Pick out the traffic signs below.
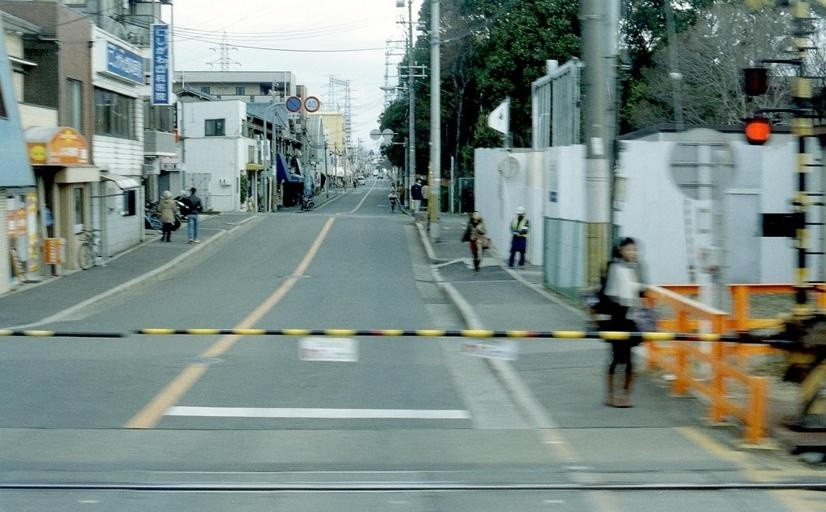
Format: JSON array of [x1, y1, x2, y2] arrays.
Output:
[[304, 96, 319, 112]]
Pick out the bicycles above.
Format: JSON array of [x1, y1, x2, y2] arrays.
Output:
[[76, 229, 104, 270]]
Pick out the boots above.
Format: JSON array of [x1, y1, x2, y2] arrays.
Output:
[[473, 259, 481, 272], [160, 227, 172, 242], [606, 373, 634, 408]]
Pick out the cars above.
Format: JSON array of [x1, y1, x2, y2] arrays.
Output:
[[373, 169, 383, 179]]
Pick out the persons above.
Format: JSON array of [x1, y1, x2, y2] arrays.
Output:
[[463, 211, 490, 271], [184, 188, 204, 243], [389, 191, 397, 213], [399, 183, 404, 205], [508, 206, 530, 267], [158, 191, 178, 242], [589, 236, 650, 409], [411, 178, 424, 211], [353, 181, 356, 189]]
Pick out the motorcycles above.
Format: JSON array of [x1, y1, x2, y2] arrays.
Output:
[[300, 195, 315, 210]]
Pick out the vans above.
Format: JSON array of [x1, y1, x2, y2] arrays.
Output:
[[357, 176, 365, 185]]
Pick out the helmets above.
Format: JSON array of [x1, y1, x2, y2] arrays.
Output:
[[515, 206, 526, 214]]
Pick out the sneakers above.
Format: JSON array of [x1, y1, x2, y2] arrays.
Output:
[[187, 238, 200, 244]]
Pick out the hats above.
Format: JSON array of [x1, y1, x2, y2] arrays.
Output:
[[162, 190, 171, 199]]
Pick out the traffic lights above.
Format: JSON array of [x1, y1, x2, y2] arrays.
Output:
[[745, 118, 770, 145], [744, 68, 768, 97]]
